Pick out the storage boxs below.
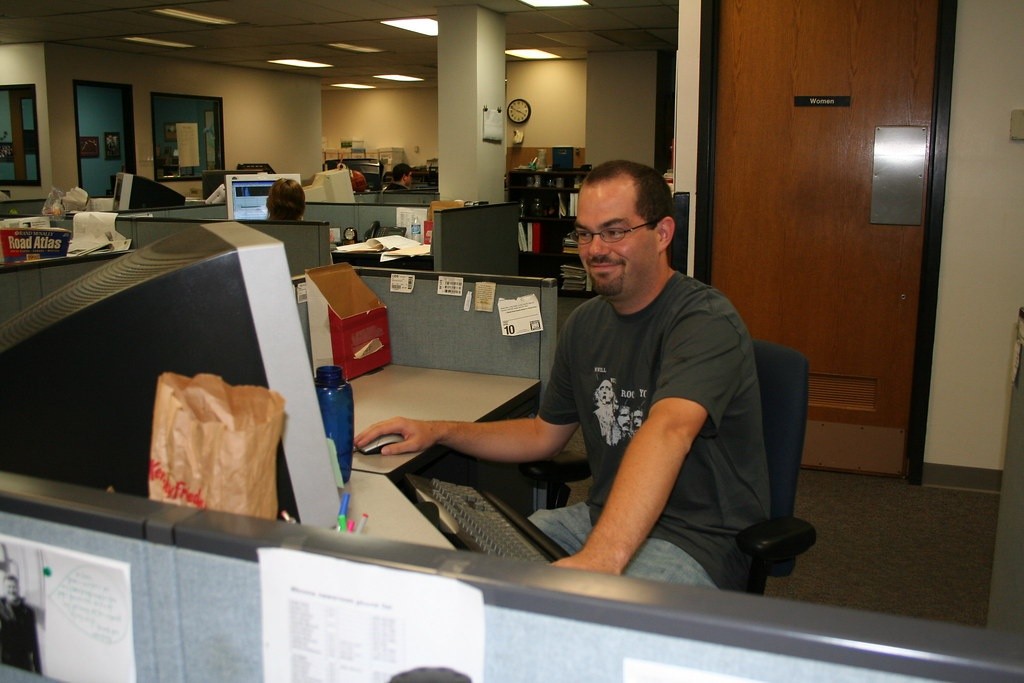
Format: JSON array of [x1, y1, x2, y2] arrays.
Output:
[[322, 137, 404, 172], [304, 263, 391, 381], [424, 200, 463, 244]]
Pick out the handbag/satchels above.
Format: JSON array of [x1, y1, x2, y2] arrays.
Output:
[[149, 371, 285, 520]]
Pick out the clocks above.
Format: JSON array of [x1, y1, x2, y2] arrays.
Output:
[[507, 99, 531, 124]]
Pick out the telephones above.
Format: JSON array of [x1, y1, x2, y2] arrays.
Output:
[[365, 221, 406, 240]]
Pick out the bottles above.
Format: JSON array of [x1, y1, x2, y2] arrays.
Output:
[[90, 200, 95, 211], [411, 216, 421, 243], [527, 177, 534, 186], [556, 178, 563, 187], [536, 149, 547, 169]]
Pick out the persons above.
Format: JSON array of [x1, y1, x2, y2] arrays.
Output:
[[0, 576, 43, 676], [266, 178, 306, 221], [107, 136, 117, 154], [387, 163, 413, 190], [353, 160, 771, 593]]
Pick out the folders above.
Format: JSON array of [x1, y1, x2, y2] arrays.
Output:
[[518, 222, 540, 252]]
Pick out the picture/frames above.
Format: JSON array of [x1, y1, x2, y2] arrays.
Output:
[[104, 132, 120, 159], [79, 137, 99, 157], [164, 122, 177, 141], [0, 142, 14, 161]]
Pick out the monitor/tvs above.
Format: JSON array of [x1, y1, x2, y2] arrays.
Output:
[[0, 168, 355, 532]]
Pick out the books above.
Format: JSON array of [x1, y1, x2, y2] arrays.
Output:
[[560, 264, 592, 291], [518, 222, 542, 253], [562, 238, 579, 254], [570, 193, 578, 216]]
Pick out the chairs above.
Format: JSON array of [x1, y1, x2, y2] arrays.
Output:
[[518, 339, 816, 596]]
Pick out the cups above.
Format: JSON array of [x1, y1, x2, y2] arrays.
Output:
[[313, 365, 354, 484], [518, 166, 529, 169]]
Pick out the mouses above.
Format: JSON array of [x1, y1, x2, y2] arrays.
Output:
[[357, 433, 404, 454]]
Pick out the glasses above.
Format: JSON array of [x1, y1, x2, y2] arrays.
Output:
[[567, 215, 667, 245]]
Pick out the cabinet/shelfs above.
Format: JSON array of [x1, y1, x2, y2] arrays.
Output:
[[508, 166, 591, 291]]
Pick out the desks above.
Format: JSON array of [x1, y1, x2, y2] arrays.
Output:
[[338, 364, 542, 550]]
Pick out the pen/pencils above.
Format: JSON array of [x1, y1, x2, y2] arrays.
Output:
[[531, 155, 537, 165], [335, 491, 371, 533], [280, 510, 292, 520]]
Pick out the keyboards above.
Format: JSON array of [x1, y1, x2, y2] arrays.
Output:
[[403, 473, 551, 565]]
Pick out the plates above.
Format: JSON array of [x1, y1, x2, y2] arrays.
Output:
[[514, 168, 532, 171]]
